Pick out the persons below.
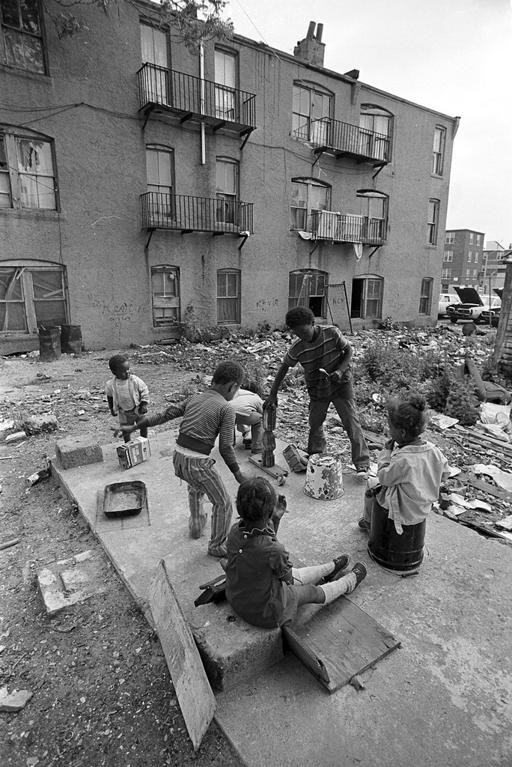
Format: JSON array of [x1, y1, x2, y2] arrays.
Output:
[[104, 354, 151, 443], [228, 378, 266, 453], [109, 360, 248, 559], [358, 392, 452, 536], [262, 304, 372, 476], [224, 474, 368, 629]]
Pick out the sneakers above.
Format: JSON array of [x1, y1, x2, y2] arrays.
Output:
[[358, 519, 372, 535], [325, 552, 353, 584], [356, 464, 371, 478], [343, 560, 368, 594]]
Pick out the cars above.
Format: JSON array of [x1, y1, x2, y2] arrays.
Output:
[[438, 286, 501, 324]]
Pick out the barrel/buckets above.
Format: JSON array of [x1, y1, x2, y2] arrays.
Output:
[[304, 452, 345, 501], [61, 323, 83, 355], [39, 326, 61, 360], [367, 503, 430, 577]]
